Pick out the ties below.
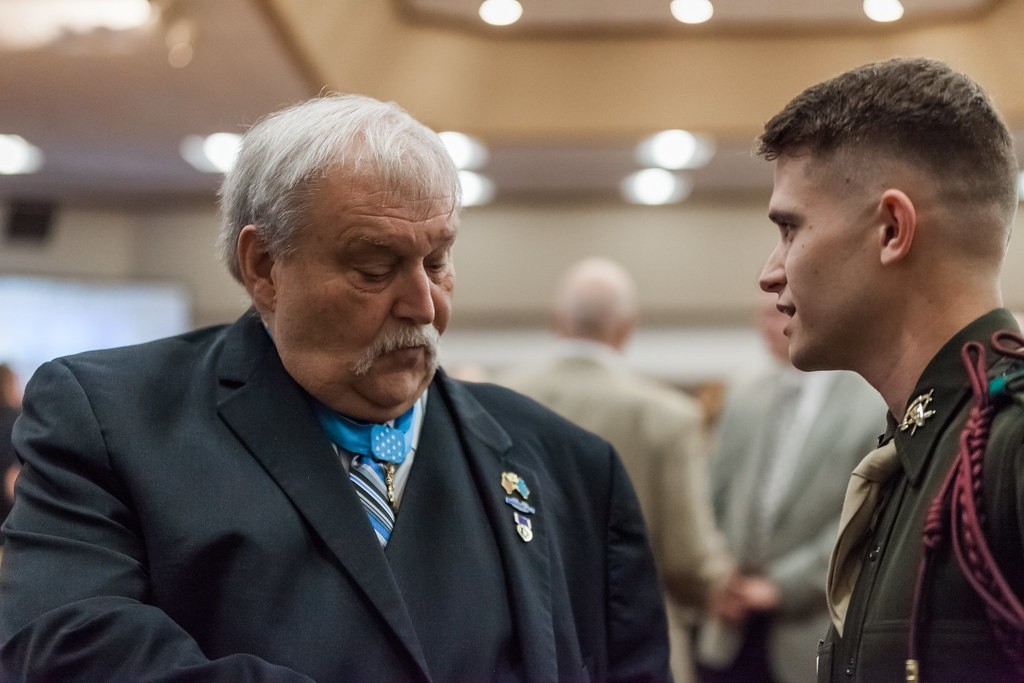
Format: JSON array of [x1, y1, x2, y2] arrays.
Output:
[[349, 452, 398, 551], [824, 436, 899, 636]]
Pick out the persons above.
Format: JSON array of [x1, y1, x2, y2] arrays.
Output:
[[521, 258, 888, 683], [749, 56, 1024, 683], [0, 93, 675, 683], [0, 363, 29, 533]]
